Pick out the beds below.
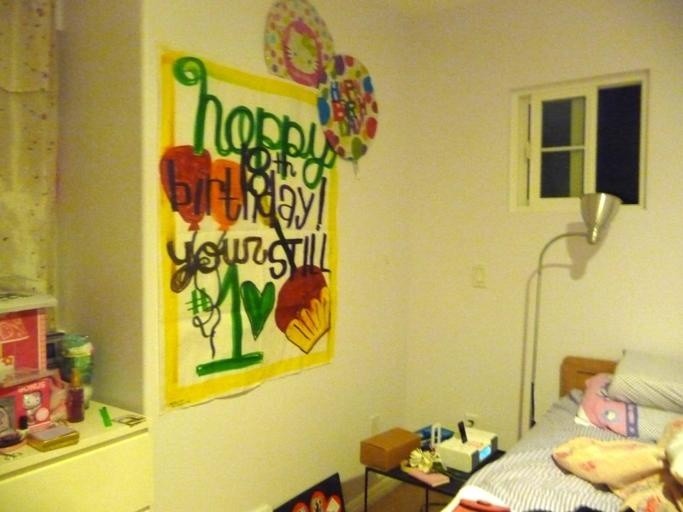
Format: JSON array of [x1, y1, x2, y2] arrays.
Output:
[[441, 346, 683, 512]]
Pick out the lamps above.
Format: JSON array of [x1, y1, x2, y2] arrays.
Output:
[[530, 193, 622, 429]]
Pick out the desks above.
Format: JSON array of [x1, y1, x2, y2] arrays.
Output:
[[364, 423, 506, 512]]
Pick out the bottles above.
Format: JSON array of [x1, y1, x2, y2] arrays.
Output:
[[68, 367, 86, 422]]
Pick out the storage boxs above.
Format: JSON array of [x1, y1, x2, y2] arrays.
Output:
[[360, 427, 421, 471]]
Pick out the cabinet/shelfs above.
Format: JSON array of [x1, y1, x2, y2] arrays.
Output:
[[0, 398, 156, 512]]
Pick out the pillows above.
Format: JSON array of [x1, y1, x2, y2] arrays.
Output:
[[573, 372, 682, 440], [605, 349, 682, 414]]
